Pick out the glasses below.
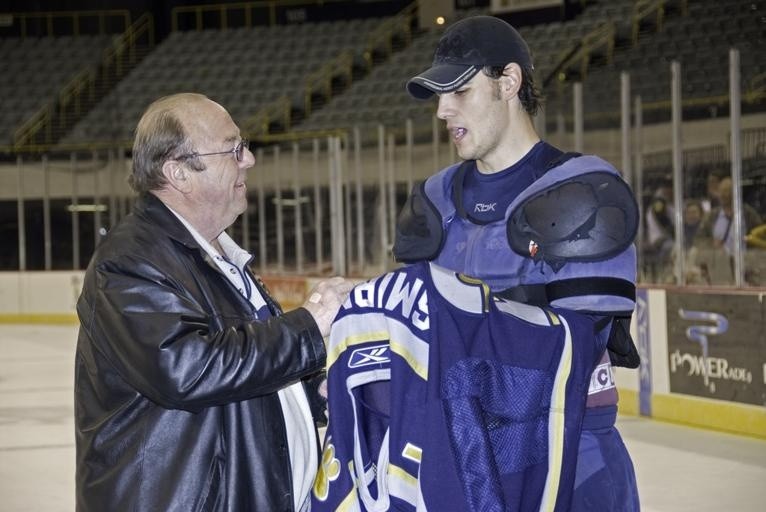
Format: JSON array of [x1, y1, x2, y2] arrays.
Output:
[[175, 137, 249, 162]]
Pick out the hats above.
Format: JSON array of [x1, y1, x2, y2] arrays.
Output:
[[408, 14, 533, 99]]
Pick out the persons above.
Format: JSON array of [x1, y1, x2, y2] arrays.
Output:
[[306, 16, 640, 512], [642, 162, 766, 287], [71, 90, 356, 511]]
